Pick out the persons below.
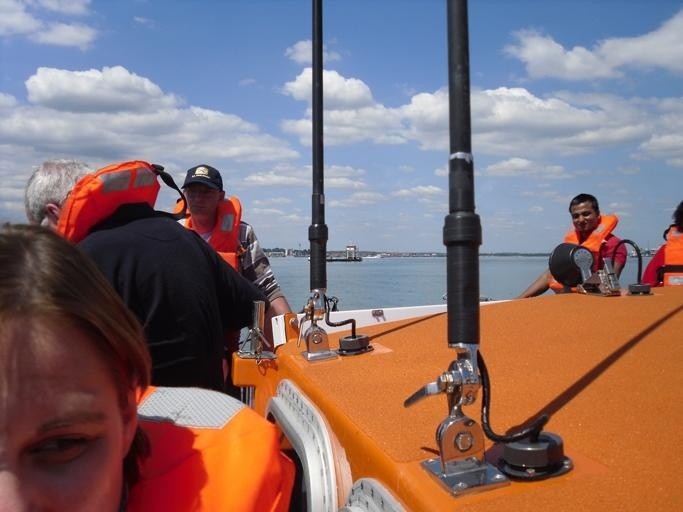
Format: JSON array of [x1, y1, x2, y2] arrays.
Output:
[[641, 200, 683, 287], [172, 164, 293, 320], [26, 157, 271, 392], [0, 222, 299, 511], [517, 192, 628, 299]]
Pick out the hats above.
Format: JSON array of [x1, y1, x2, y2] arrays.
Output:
[[181, 164, 223, 190]]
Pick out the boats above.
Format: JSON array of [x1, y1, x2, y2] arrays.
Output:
[[228, 284, 681, 512]]
